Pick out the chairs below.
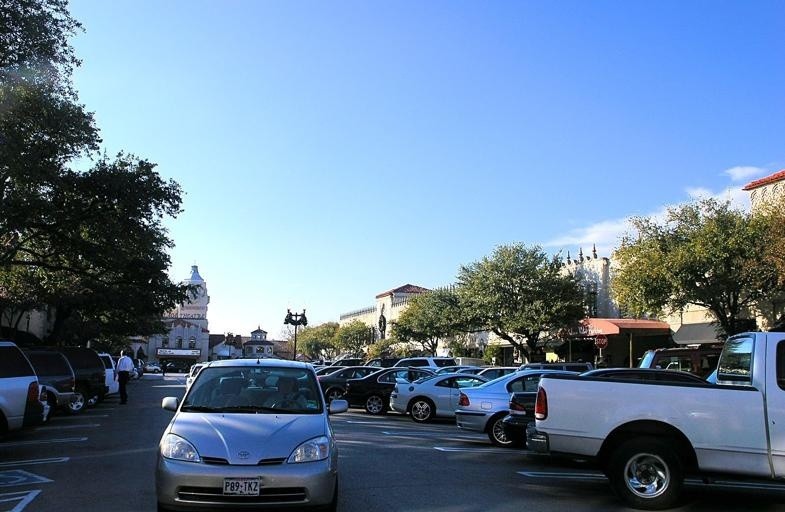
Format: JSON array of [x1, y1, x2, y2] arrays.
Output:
[[205, 376, 312, 407]]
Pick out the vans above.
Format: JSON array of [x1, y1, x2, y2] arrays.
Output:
[[636, 343, 726, 380]]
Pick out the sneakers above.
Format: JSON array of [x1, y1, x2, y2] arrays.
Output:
[[119, 400, 128, 405]]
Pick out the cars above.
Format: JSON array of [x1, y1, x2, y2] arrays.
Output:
[[0, 338, 145, 441], [501, 365, 715, 473], [144, 362, 161, 374]]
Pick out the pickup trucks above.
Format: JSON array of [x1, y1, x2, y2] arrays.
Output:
[[522, 330, 785, 511]]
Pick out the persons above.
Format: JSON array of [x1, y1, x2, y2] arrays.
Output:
[[160, 361, 167, 377], [113, 348, 135, 406]]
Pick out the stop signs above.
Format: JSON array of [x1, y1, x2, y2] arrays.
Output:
[[593, 333, 608, 349]]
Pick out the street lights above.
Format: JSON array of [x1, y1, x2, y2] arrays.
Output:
[[283, 308, 307, 359]]
